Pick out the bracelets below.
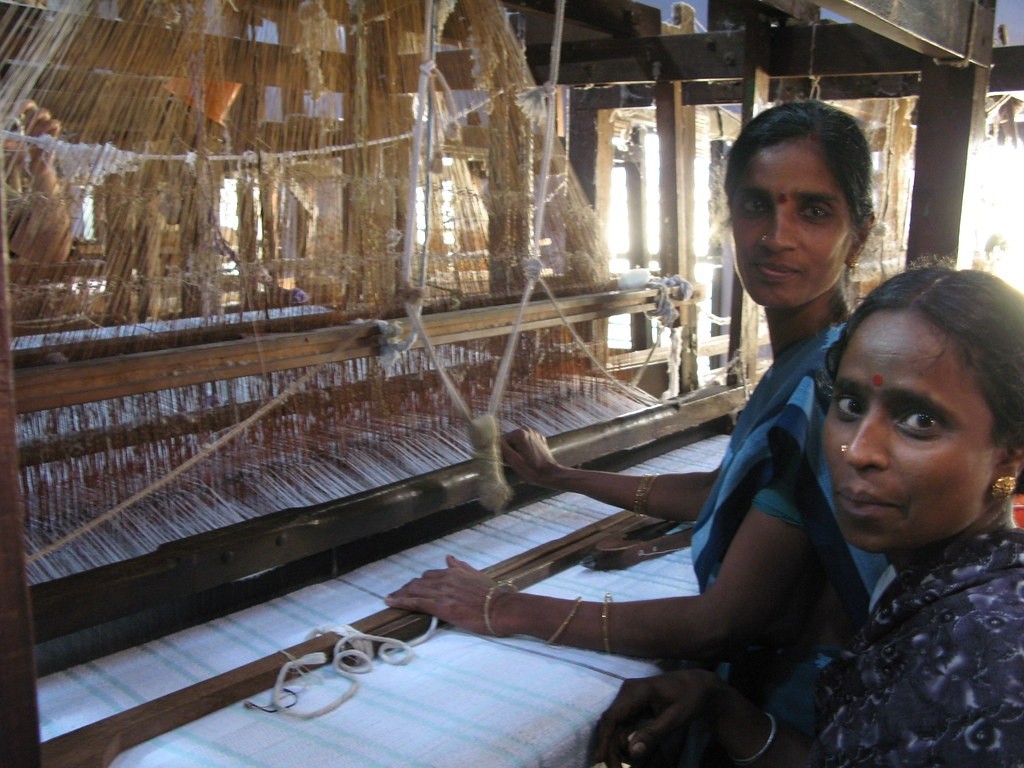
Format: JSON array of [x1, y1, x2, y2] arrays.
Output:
[[601, 594, 613, 655], [634, 473, 660, 517], [483, 580, 517, 638], [544, 596, 582, 645], [730, 711, 777, 766]]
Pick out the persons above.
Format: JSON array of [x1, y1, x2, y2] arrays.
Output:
[[383, 103, 894, 666], [0, 100, 72, 265], [584, 266, 1024, 768]]
